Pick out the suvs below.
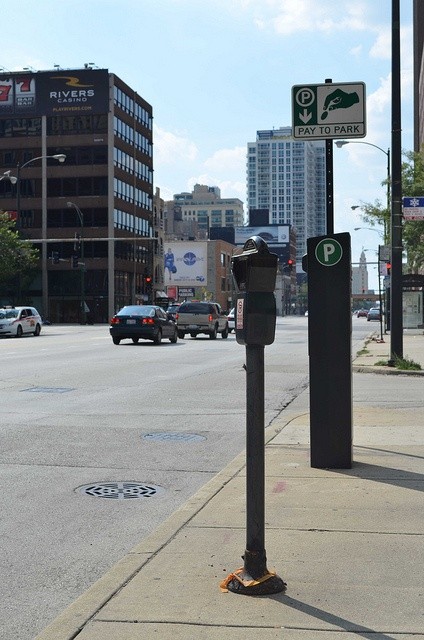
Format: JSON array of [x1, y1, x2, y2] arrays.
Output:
[[175, 300, 228, 339]]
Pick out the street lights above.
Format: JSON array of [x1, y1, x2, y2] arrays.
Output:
[[351, 205, 386, 331], [334, 141, 390, 334], [66, 202, 85, 324], [16, 154, 66, 307]]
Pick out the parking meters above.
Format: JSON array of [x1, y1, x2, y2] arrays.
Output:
[[225, 235, 287, 595]]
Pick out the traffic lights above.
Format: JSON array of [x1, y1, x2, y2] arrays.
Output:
[[386, 263, 391, 274], [362, 287, 365, 293], [144, 267, 152, 282], [287, 260, 293, 271]]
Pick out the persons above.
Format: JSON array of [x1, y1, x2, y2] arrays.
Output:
[[165, 248, 174, 281]]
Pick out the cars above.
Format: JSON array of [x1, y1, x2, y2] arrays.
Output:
[[228, 307, 236, 332], [109, 305, 178, 345], [367, 310, 381, 321], [357, 310, 367, 318], [0, 305, 42, 337]]
